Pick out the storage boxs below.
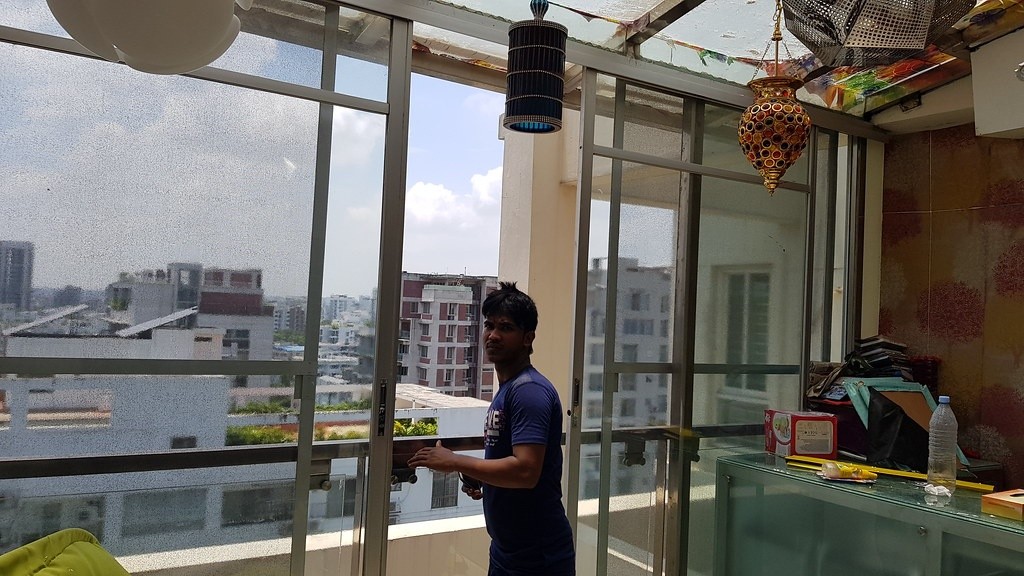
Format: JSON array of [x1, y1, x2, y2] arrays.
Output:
[[764, 410, 838, 459]]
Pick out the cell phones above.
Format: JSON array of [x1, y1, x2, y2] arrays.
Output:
[[462, 473, 481, 494]]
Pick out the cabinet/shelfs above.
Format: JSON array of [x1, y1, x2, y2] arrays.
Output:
[[714, 453, 1024, 576]]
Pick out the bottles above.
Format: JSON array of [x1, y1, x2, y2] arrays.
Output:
[[927, 396, 958, 495]]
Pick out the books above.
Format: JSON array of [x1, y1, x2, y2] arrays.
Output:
[[858, 334, 913, 376]]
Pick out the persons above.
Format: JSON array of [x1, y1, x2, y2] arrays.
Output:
[[407, 283, 577, 576]]
